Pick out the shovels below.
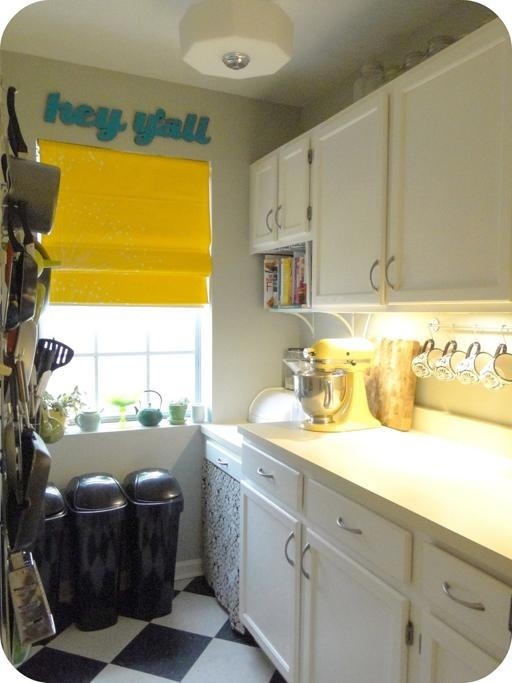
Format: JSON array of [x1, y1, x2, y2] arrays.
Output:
[[32, 338, 74, 417]]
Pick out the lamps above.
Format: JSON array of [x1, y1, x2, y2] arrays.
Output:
[[176, 0, 295, 81]]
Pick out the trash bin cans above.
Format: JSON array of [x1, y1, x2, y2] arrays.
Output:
[[30, 468, 185, 632]]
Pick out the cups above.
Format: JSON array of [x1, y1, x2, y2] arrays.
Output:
[[168, 420, 187, 425], [169, 405, 188, 419], [411, 339, 512, 391], [192, 406, 205, 424]]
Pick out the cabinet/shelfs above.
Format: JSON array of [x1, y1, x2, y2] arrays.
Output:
[[414, 510, 512, 683], [247, 133, 314, 254], [238, 424, 413, 682], [311, 15, 512, 307], [200, 425, 242, 633]]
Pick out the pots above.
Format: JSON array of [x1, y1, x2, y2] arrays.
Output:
[[1, 97, 61, 667]]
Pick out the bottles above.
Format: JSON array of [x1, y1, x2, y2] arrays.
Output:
[[284, 348, 304, 391]]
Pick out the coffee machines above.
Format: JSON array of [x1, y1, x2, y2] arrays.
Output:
[[296, 338, 386, 433]]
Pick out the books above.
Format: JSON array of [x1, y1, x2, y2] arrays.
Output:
[[262, 244, 306, 308]]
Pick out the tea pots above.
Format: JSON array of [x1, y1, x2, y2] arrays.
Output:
[[133, 389, 163, 426], [75, 408, 105, 433]]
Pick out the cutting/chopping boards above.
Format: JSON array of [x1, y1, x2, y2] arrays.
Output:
[[373, 337, 420, 433]]
